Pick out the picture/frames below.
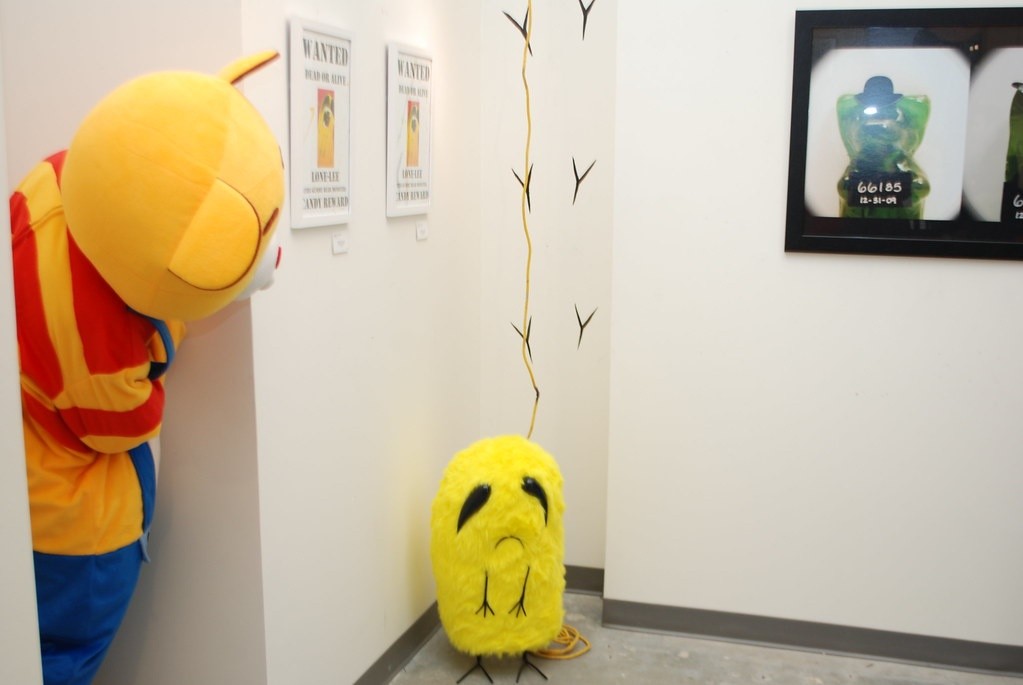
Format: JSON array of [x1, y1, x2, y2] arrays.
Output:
[[386, 46, 435, 218], [286, 19, 354, 231], [785, 7, 1023, 260]]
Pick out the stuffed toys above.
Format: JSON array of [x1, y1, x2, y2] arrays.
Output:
[[432, 433, 566, 654], [4, 49, 289, 685]]
